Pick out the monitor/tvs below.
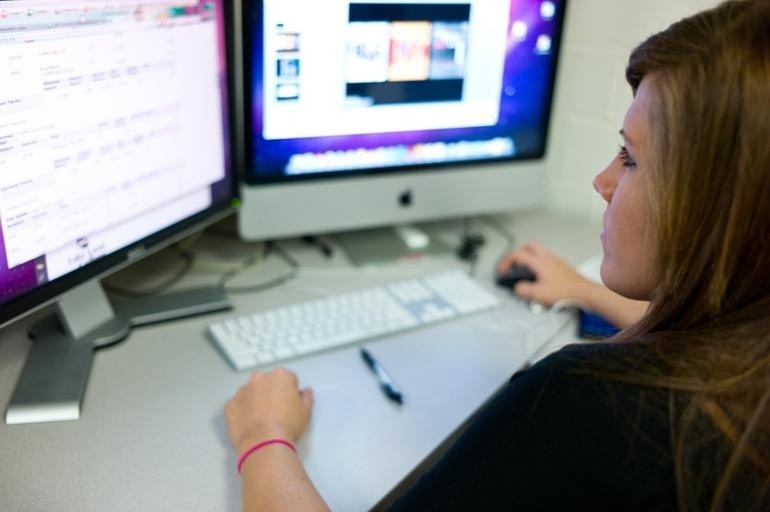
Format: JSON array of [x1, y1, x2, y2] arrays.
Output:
[[0, 1, 238, 423], [238, 1, 571, 267]]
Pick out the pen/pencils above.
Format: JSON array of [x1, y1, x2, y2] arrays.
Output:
[[360, 347, 402, 404]]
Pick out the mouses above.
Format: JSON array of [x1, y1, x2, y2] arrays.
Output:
[[495, 265, 536, 289]]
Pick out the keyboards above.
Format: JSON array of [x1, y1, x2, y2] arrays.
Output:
[[206, 270, 502, 373]]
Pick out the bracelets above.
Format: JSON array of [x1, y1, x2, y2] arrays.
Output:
[[238, 437, 296, 473]]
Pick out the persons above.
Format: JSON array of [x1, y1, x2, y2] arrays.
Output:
[[220, 0, 770, 512]]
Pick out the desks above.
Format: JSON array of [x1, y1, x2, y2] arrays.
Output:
[[1, 203, 603, 512]]
